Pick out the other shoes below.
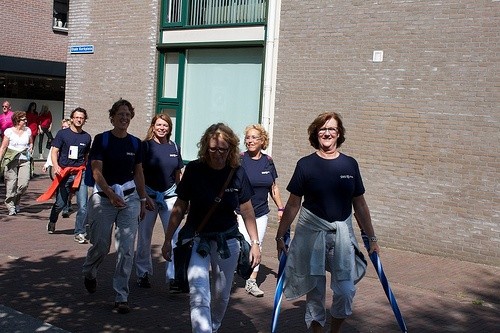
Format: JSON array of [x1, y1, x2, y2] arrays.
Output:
[[46, 222, 55, 234], [68, 205, 73, 212], [83, 276, 97, 294], [245, 279, 264, 297], [115, 301, 130, 314], [137, 272, 150, 284], [62, 210, 69, 217]]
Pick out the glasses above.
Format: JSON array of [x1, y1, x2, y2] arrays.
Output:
[[1, 105, 9, 108], [115, 110, 133, 118], [206, 146, 231, 154], [18, 117, 28, 122], [316, 126, 338, 135]]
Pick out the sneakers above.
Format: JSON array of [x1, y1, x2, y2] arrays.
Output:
[[74, 234, 87, 244], [15, 205, 21, 213], [169, 281, 182, 294], [8, 208, 17, 216]]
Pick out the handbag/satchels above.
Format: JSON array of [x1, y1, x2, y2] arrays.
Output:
[[173, 240, 194, 294], [1, 148, 23, 171]]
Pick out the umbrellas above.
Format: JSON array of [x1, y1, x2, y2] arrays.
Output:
[[271, 228, 290, 333], [353, 212, 408, 333]]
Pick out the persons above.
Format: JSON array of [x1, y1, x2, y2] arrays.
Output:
[[25, 101, 53, 159], [83, 100, 146, 311], [233, 124, 284, 296], [0, 100, 14, 138], [42, 118, 73, 218], [36, 108, 92, 243], [0, 111, 33, 215], [162, 123, 261, 333], [136, 113, 183, 292], [276, 112, 379, 333]]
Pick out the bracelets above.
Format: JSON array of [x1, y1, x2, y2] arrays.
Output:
[[251, 240, 260, 245], [278, 209, 284, 211], [370, 237, 377, 241], [140, 198, 146, 201], [276, 237, 284, 241]]
